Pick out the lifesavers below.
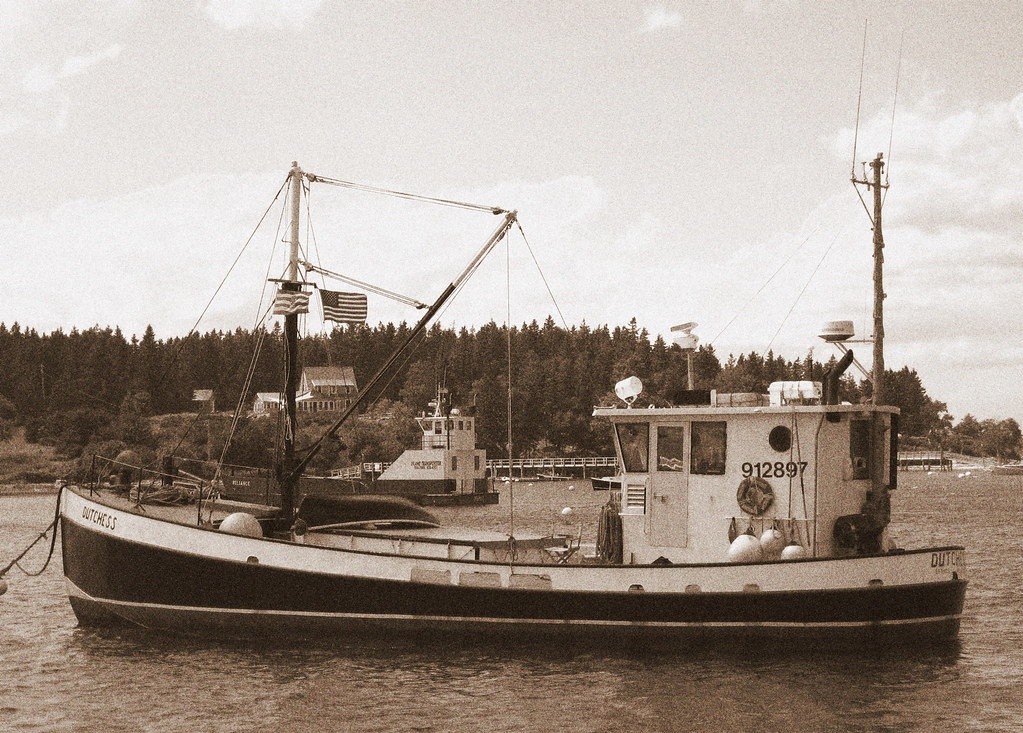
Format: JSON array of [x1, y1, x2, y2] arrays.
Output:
[[736, 476, 775, 517]]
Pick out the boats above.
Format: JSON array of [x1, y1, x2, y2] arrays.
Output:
[[57, 161, 970, 655], [590, 476, 621, 491], [370, 368, 501, 510]]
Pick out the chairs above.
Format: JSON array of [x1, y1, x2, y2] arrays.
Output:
[[543, 526, 583, 567]]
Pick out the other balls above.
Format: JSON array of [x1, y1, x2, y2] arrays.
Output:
[[219, 513, 263, 537]]
